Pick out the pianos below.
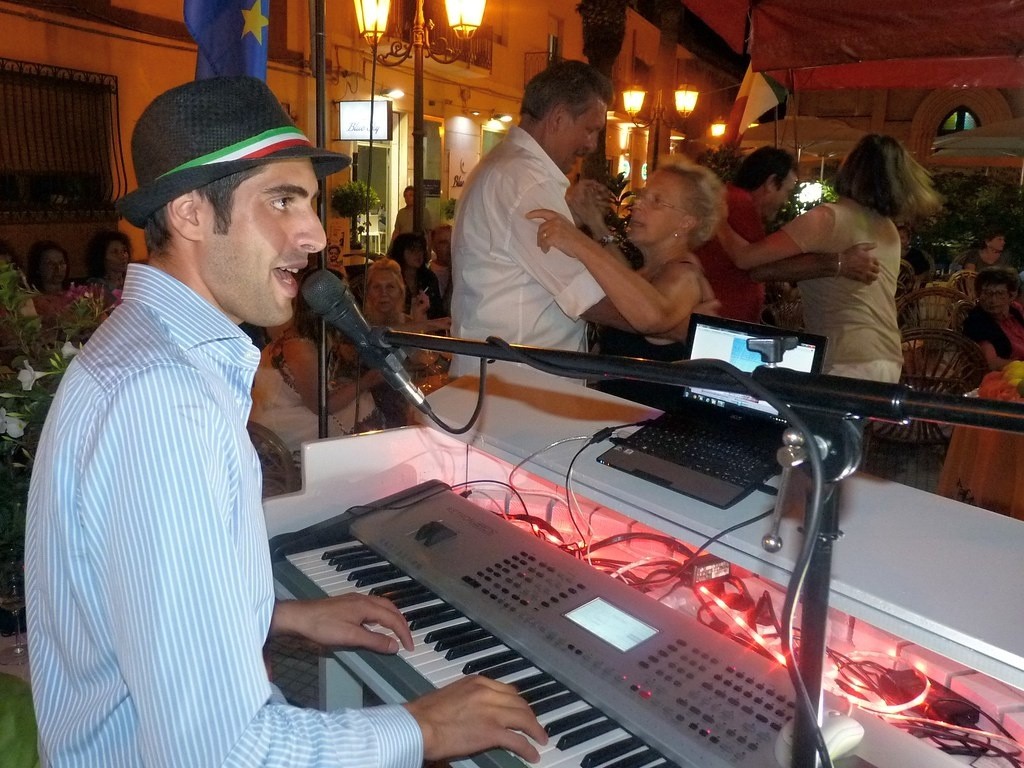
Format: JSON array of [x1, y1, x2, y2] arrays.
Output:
[[267, 476, 868, 767]]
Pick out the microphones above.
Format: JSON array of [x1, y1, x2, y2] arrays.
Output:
[[299, 270, 433, 414]]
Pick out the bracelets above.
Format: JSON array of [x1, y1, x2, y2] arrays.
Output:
[[835, 253, 841, 278]]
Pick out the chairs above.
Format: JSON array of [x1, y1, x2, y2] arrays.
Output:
[[244, 257, 1024, 500]]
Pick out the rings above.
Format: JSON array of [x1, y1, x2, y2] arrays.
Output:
[[544, 232, 547, 238]]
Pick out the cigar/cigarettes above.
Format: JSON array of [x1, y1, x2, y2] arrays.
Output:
[[423, 287, 428, 293]]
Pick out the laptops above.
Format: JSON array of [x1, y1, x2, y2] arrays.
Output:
[[598, 311, 828, 510]]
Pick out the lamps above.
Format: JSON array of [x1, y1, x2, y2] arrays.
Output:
[[381, 89, 403, 101], [489, 112, 512, 121]]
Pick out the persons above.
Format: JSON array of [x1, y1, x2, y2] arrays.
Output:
[[250, 187, 452, 466], [695, 146, 879, 329], [526, 154, 730, 361], [24, 78, 550, 768], [962, 264, 1024, 372], [897, 224, 930, 276], [27, 240, 70, 291], [84, 231, 130, 288], [963, 228, 1008, 292], [715, 133, 946, 384], [450, 58, 721, 388]]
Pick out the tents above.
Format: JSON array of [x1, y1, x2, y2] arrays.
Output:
[[680, 0, 1024, 165]]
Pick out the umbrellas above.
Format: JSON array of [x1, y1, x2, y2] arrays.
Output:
[[928, 116, 1024, 188], [740, 117, 870, 182]]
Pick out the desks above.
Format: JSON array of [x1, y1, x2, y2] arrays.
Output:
[[938, 360, 1024, 522]]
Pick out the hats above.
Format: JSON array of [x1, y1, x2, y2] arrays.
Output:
[[114, 75, 352, 228]]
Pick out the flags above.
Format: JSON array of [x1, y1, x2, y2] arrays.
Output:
[[723, 60, 787, 144], [183, 0, 269, 83]]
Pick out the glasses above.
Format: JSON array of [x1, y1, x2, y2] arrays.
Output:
[[631, 185, 691, 218], [982, 288, 1009, 298]]
